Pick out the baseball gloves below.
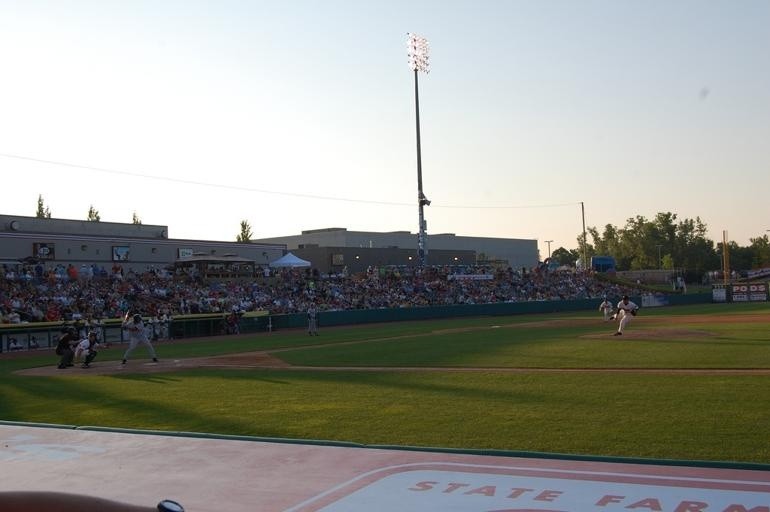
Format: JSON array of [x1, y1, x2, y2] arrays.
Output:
[[631, 309, 637, 316], [100, 343, 112, 349]]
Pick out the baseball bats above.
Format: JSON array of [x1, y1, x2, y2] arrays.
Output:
[[122, 310, 130, 327]]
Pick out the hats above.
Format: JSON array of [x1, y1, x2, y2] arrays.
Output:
[[134, 313, 142, 323], [622, 295, 630, 299], [68, 327, 76, 333]]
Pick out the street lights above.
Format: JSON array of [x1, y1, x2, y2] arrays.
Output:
[[406, 31, 433, 267]]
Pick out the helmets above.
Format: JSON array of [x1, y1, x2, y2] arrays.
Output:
[[88, 331, 98, 337]]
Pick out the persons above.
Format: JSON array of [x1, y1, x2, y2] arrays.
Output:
[[118, 307, 160, 365], [55, 327, 77, 369], [75, 331, 109, 369]]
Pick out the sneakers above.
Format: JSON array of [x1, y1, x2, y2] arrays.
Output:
[[57, 362, 90, 369], [123, 359, 126, 363], [615, 331, 622, 336], [153, 357, 159, 362]]
